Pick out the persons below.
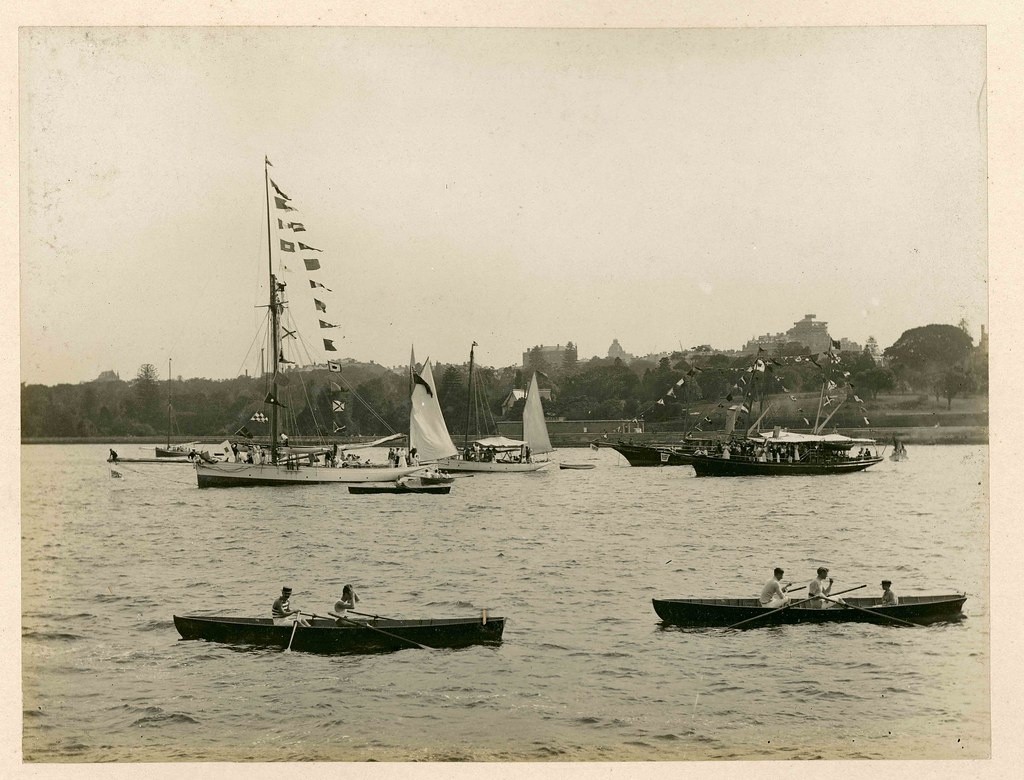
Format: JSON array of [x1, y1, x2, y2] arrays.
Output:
[[231, 442, 266, 465], [809, 567, 851, 608], [472, 443, 497, 462], [272, 587, 311, 627], [628, 437, 633, 445], [279, 433, 289, 446], [617, 437, 624, 445], [526, 446, 530, 463], [716, 439, 723, 454], [729, 437, 742, 452], [389, 446, 420, 467], [747, 440, 808, 462], [334, 584, 372, 627], [325, 450, 361, 469], [759, 568, 788, 608], [110, 448, 118, 459], [188, 449, 198, 460], [858, 447, 871, 459], [872, 580, 898, 606]]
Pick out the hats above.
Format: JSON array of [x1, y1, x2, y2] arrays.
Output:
[[880, 581, 893, 585], [282, 587, 292, 594]]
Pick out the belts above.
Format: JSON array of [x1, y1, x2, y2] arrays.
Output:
[[762, 603, 766, 604]]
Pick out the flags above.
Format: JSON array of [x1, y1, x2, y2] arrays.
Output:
[[234, 159, 346, 438]]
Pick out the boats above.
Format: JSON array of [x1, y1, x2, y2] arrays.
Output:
[[891, 451, 908, 463], [347, 486, 450, 494], [171, 611, 505, 652], [560, 464, 595, 469], [666, 596, 968, 625]]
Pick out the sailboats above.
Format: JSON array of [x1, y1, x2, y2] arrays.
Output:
[[155, 356, 196, 457], [394, 341, 475, 487], [590, 363, 886, 478], [496, 370, 550, 463], [437, 341, 552, 472], [107, 153, 432, 487]]
